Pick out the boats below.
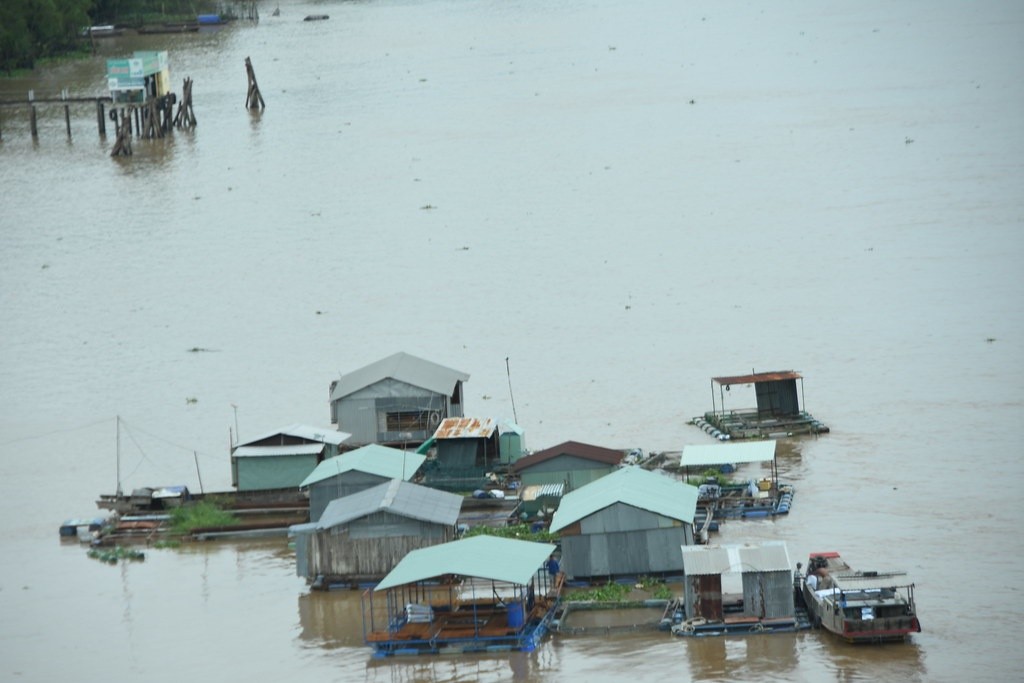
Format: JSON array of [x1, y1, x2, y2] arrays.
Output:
[[804, 551, 921, 642]]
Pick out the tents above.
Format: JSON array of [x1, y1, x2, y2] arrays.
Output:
[[711, 370, 805, 423], [680, 439, 777, 483], [374, 533, 557, 636]]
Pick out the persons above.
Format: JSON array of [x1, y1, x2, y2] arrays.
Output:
[[793, 562, 805, 600]]
